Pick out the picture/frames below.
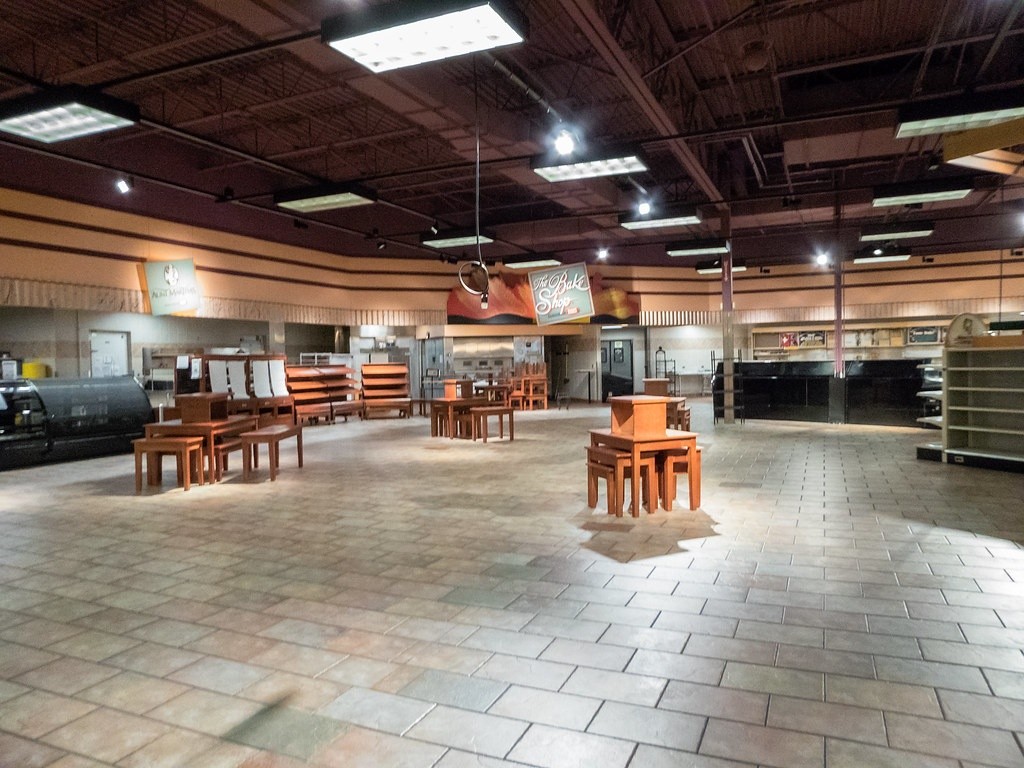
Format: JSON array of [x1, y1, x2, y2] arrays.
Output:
[[613, 348, 624, 363], [601, 348, 608, 363]]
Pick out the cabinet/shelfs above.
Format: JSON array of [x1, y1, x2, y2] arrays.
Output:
[[914, 347, 1024, 474], [711, 349, 745, 426]]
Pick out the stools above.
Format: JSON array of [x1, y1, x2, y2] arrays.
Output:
[[130, 391, 303, 492], [584, 378, 702, 519], [428, 363, 548, 443]]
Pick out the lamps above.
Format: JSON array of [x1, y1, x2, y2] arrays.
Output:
[[871, 173, 974, 209], [856, 220, 934, 242], [694, 258, 748, 276], [853, 247, 912, 264], [892, 91, 1024, 139], [420, 225, 495, 250], [0, 82, 142, 144], [532, 142, 654, 183], [665, 239, 731, 258], [274, 181, 381, 215], [618, 207, 704, 231], [501, 253, 563, 270], [320, 0, 531, 73]]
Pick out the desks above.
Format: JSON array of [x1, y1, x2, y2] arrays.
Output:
[[142, 414, 260, 487], [585, 428, 700, 518], [667, 396, 687, 430], [429, 396, 487, 439]]
